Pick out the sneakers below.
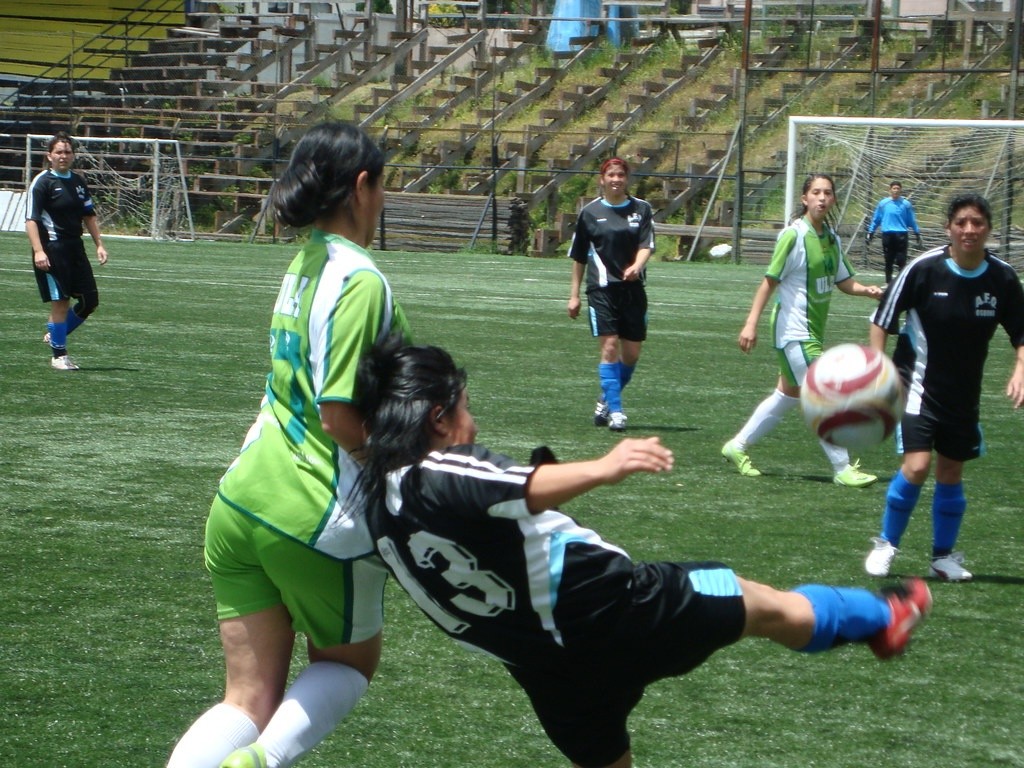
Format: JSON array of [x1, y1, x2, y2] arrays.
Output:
[[51, 354, 80, 371], [865, 535, 902, 577], [929, 551, 973, 581], [607, 410, 628, 430], [869, 576, 932, 661], [832, 458, 878, 489], [593, 400, 610, 424], [43, 333, 52, 344], [721, 440, 761, 477]]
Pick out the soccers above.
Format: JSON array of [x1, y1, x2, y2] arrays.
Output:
[[798, 343, 907, 450]]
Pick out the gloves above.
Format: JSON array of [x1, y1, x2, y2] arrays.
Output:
[[917, 233, 923, 245], [865, 232, 873, 246]]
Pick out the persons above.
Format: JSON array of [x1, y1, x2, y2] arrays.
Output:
[[337, 343, 931, 767], [165, 121, 416, 768], [866, 182, 923, 290], [720, 173, 884, 488], [24, 133, 108, 370], [567, 156, 655, 432], [865, 193, 1024, 579]]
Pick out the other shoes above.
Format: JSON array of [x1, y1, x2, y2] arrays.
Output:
[[880, 282, 892, 290]]
